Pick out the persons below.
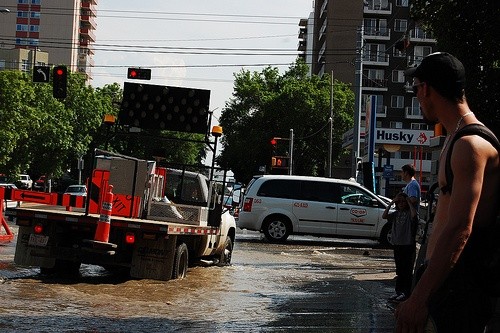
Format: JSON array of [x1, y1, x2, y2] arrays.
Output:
[[393, 52, 500, 333], [175, 182, 199, 200], [400, 164, 421, 220], [382, 192, 420, 302]]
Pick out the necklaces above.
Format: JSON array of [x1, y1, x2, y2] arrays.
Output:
[[439, 112, 474, 160]]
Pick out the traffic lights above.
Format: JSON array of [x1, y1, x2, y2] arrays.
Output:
[[53, 65, 67, 99], [127, 67, 152, 81], [276, 158, 289, 167], [271, 139, 278, 156]]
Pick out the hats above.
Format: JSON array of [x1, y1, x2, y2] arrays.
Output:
[[404, 52, 466, 89]]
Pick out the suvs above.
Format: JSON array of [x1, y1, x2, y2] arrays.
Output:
[[236, 174, 398, 249]]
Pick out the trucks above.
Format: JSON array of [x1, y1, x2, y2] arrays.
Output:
[[5, 115, 242, 282]]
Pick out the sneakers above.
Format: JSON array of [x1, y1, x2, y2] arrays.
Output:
[[388, 294, 401, 301], [394, 292, 411, 302]]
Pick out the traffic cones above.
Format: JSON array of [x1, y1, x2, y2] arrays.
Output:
[[83, 185, 117, 249]]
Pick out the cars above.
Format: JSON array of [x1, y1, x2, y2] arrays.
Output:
[[342, 193, 395, 209], [416, 194, 438, 244], [16, 174, 33, 190], [0, 183, 23, 212], [34, 176, 49, 189], [63, 184, 88, 196]]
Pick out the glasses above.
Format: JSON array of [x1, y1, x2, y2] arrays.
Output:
[[412, 82, 432, 95]]
